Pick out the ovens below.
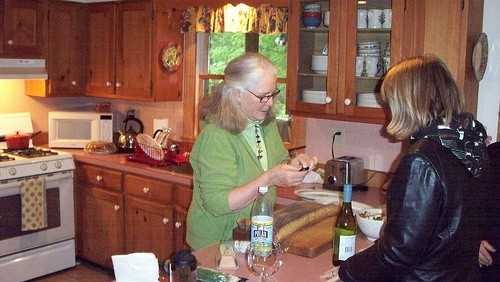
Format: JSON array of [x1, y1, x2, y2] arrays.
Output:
[[0, 169, 76, 282]]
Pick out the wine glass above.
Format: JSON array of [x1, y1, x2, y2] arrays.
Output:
[[247, 240, 283, 282]]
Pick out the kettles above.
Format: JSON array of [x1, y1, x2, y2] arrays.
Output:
[[117, 114, 144, 152]]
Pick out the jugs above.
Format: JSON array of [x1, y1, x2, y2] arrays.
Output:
[[276, 115, 292, 144], [164, 249, 201, 282], [153, 128, 176, 151], [357, 8, 392, 29]]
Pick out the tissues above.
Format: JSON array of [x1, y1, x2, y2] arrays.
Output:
[[110, 252, 170, 282]]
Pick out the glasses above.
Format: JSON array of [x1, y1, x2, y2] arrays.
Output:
[[245, 87, 280, 103]]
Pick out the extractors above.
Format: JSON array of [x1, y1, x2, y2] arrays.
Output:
[[0, 58, 48, 80]]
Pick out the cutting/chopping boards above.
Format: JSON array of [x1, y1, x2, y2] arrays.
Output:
[[272, 200, 342, 258]]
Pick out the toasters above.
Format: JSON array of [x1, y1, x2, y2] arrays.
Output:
[[324, 156, 364, 190]]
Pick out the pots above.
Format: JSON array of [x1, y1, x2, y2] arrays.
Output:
[[0, 132, 30, 150]]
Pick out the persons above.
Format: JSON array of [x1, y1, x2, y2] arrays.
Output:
[[477, 140, 500, 282], [185, 52, 318, 253], [320, 54, 489, 282]]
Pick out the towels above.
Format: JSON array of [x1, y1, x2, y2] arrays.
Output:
[[19, 176, 48, 231]]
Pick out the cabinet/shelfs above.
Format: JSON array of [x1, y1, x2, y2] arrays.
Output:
[[0, 0, 184, 102], [284, 0, 485, 126], [74, 160, 193, 274]]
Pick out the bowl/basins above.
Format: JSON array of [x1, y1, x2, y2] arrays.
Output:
[[355, 208, 386, 241], [356, 42, 390, 77], [302, 4, 322, 28]]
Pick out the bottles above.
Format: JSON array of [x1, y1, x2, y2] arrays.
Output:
[[332, 184, 357, 266], [250, 186, 274, 258], [233, 217, 251, 254]]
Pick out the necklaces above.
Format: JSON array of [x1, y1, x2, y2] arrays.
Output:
[[255, 125, 263, 160]]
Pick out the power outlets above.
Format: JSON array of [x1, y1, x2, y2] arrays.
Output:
[[328, 126, 345, 146]]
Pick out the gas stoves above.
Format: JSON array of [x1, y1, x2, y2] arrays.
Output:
[[0, 112, 76, 181]]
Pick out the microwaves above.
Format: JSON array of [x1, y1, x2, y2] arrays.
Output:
[[48, 109, 113, 148]]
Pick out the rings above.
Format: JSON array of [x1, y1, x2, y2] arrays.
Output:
[[332, 271, 334, 276]]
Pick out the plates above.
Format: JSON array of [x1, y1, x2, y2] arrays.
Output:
[[302, 90, 327, 104], [136, 134, 164, 161], [161, 41, 183, 72], [294, 189, 372, 212], [356, 92, 385, 107], [311, 44, 328, 74]]
